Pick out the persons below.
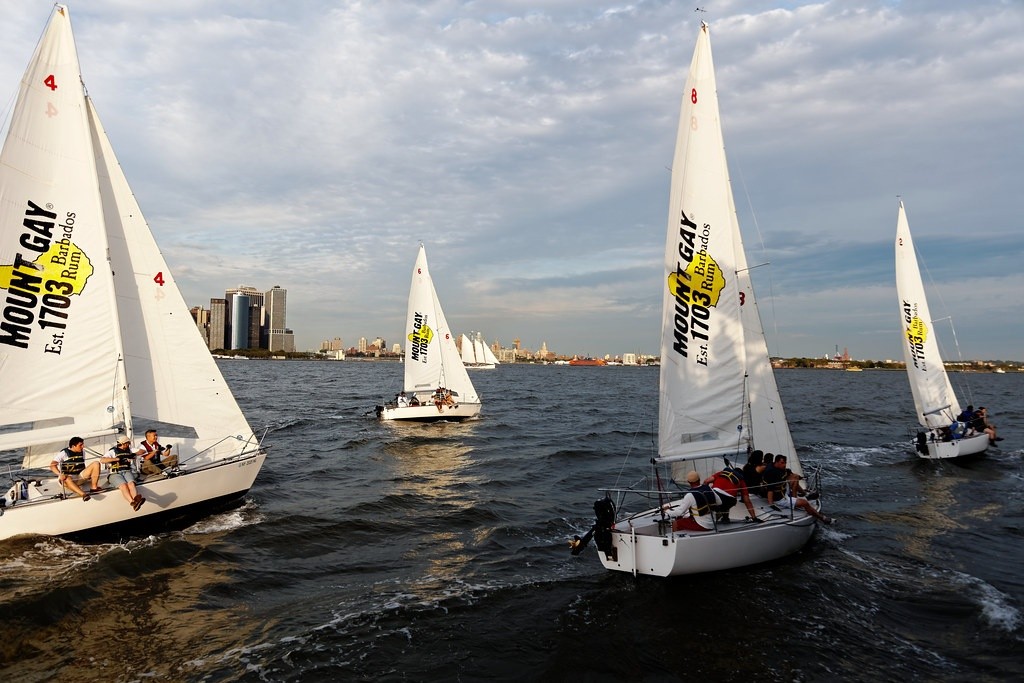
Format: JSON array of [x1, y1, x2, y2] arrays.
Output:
[[665, 449, 836, 531], [394, 392, 419, 406], [431, 390, 458, 413], [101, 436, 146, 511], [942, 406, 1005, 448], [50, 437, 106, 501], [136, 430, 179, 478]]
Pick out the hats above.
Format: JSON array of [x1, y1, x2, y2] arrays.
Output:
[[687, 471, 700, 482], [117, 436, 131, 444]]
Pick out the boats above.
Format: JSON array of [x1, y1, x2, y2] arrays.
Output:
[[554, 358, 648, 367]]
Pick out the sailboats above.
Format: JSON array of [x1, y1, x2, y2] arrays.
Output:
[[0, 3, 271, 548], [457, 333, 500, 370], [895, 196, 995, 461], [375, 246, 483, 424], [568, 20, 822, 578]]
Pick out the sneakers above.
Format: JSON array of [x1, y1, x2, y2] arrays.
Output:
[[993, 436, 1004, 442], [83, 493, 91, 501], [130, 494, 142, 506], [170, 469, 186, 476], [989, 442, 999, 448], [90, 488, 106, 494], [822, 516, 838, 525], [134, 498, 146, 511]]
